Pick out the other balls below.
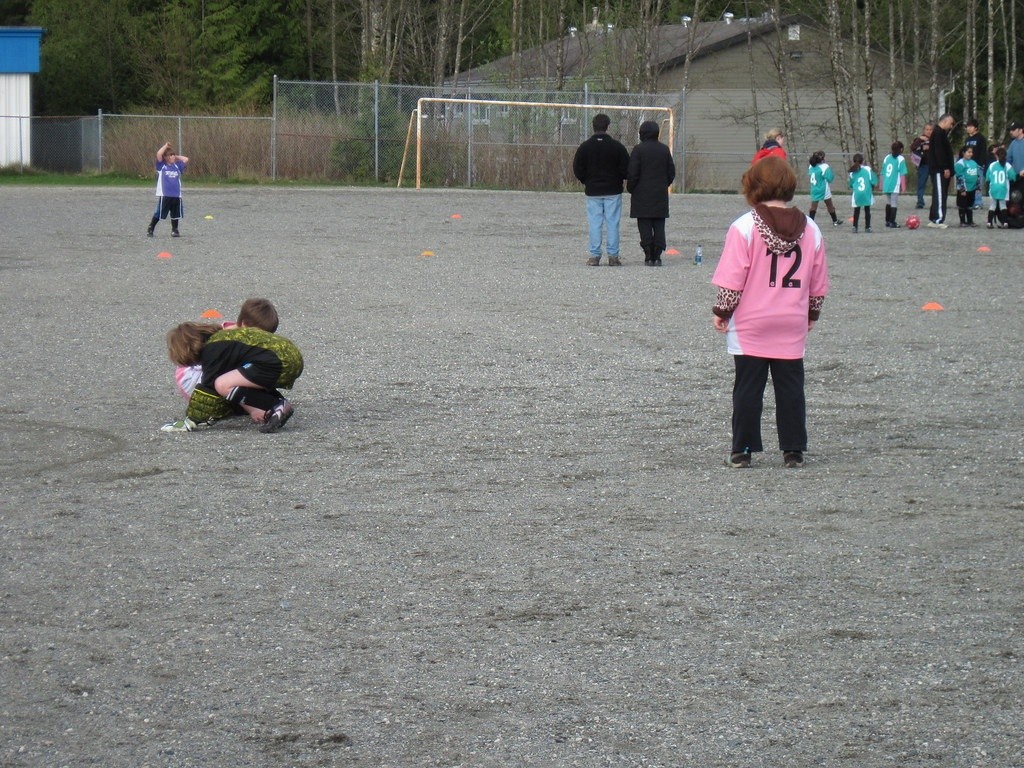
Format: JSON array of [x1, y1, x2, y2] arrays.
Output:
[[906, 215, 919, 229]]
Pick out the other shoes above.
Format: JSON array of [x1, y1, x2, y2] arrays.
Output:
[[171, 231, 179, 237], [928, 222, 949, 229], [147, 224, 154, 237], [853, 227, 857, 233], [987, 223, 994, 228], [865, 227, 872, 232], [645, 260, 653, 265], [654, 259, 661, 266], [1002, 222, 1008, 229], [968, 222, 977, 226], [890, 223, 900, 228], [961, 223, 968, 227], [886, 223, 890, 227], [917, 203, 923, 208]]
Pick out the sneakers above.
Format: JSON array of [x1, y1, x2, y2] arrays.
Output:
[[833, 219, 843, 226], [723, 450, 752, 467], [609, 256, 622, 265], [260, 399, 293, 432], [784, 451, 806, 467], [161, 421, 188, 432], [586, 257, 601, 265]]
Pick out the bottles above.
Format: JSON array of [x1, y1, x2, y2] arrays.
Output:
[[695, 245, 702, 265]]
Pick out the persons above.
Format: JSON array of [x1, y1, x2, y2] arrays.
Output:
[[910, 114, 1024, 228], [572, 113, 630, 267], [146, 142, 190, 238], [161, 321, 304, 434], [626, 121, 676, 266], [175, 298, 279, 421], [747, 128, 786, 168], [878, 141, 908, 228], [808, 151, 843, 227], [847, 153, 877, 233], [711, 157, 827, 468]]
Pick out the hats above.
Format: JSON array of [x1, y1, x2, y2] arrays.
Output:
[[1007, 121, 1023, 130]]
